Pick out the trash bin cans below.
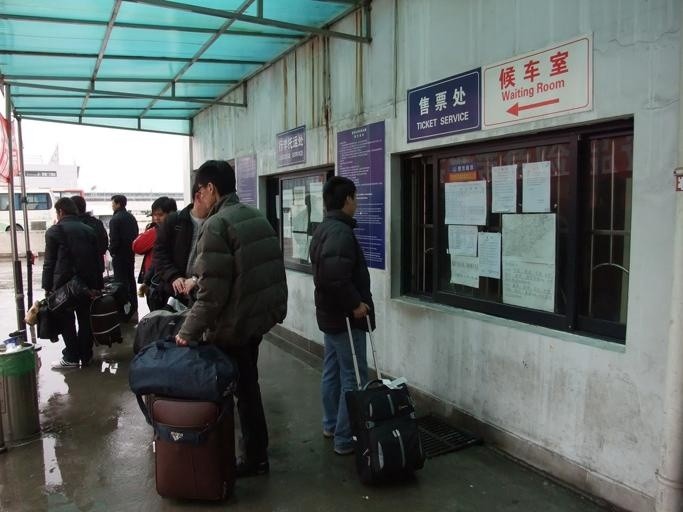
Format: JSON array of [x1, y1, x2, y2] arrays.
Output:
[[0, 341, 43, 449]]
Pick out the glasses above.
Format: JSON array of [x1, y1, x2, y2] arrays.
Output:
[[196, 185, 206, 193]]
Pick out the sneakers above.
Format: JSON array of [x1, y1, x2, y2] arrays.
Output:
[[322, 427, 352, 454], [238, 459, 272, 476], [50, 359, 91, 370]]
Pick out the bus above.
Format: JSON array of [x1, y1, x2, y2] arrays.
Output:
[[0, 187, 85, 231]]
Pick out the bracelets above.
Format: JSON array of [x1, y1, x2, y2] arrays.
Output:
[[192, 276, 197, 285]]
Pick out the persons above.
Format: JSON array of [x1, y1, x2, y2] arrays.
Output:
[[42, 196, 108, 369], [175, 160, 288, 482], [108, 195, 139, 324], [152, 183, 206, 304], [132, 196, 177, 313], [308, 176, 377, 455]]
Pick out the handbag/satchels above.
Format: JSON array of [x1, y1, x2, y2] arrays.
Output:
[[127, 308, 236, 399], [23, 278, 93, 341]]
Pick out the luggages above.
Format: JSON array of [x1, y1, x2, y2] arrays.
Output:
[[344, 308, 426, 482], [90, 275, 135, 347], [150, 395, 236, 503]]
